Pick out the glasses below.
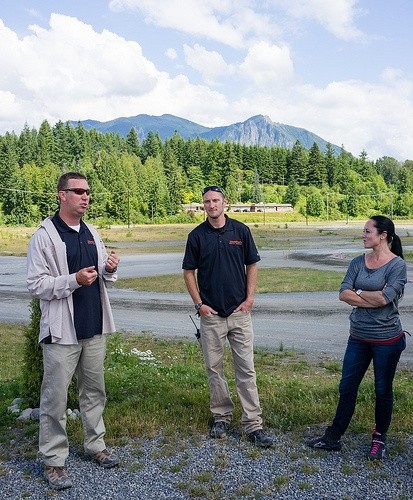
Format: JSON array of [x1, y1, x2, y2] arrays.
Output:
[[62, 189, 90, 196], [202, 186, 224, 195]]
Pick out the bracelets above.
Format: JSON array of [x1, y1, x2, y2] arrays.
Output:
[[195, 302, 202, 310], [104, 266, 116, 273], [356, 289, 362, 296]]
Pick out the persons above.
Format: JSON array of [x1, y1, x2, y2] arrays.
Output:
[[27, 173, 120, 489], [182, 185, 272, 444], [305, 215, 406, 461]]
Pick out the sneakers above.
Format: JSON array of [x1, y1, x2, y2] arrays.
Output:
[[248, 430, 273, 446], [307, 429, 341, 451], [43, 463, 72, 489], [210, 421, 228, 438], [367, 430, 386, 460], [93, 449, 120, 468]]
